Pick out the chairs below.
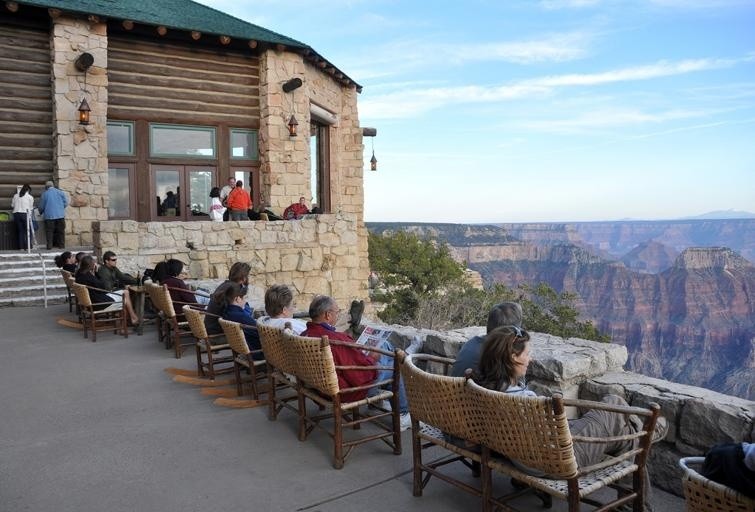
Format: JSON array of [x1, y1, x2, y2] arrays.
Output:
[[59, 269, 129, 341], [259, 212, 270, 221], [678, 453, 754, 512], [218, 316, 295, 402], [183, 304, 234, 383]]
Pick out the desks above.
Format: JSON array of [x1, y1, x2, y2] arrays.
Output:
[[128, 285, 155, 336]]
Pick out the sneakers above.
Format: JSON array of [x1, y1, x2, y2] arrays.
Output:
[[618, 500, 654, 512], [651, 415, 670, 446], [365, 400, 390, 417], [131, 317, 152, 324], [405, 336, 424, 367], [348, 298, 366, 335], [399, 412, 424, 432]]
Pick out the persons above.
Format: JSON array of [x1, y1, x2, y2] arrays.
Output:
[[284, 197, 309, 219], [209, 187, 227, 223], [441, 302, 557, 489], [226, 180, 252, 221], [701, 417, 754, 502], [11, 185, 34, 250], [470, 326, 669, 511], [37, 181, 68, 250], [219, 177, 236, 221]]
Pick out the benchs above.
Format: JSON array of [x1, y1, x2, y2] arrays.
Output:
[[397, 348, 665, 511], [256, 315, 404, 470], [144, 276, 211, 359]]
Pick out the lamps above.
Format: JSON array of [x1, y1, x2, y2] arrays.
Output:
[[77, 70, 91, 126], [370, 134, 377, 171], [286, 90, 299, 138]]
